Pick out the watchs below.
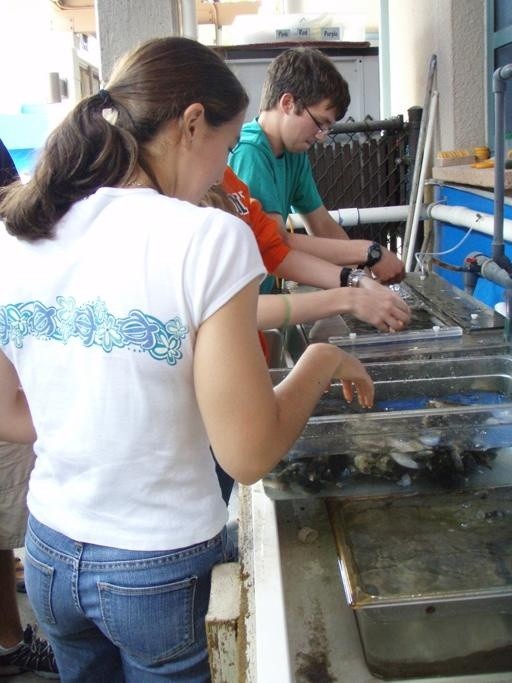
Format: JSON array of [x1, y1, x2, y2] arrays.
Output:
[[357, 241, 383, 267]]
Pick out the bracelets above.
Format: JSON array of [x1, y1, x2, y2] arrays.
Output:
[[278, 292, 291, 332], [347, 268, 369, 286], [340, 265, 351, 286]]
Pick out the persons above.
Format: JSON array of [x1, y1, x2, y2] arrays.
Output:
[[194, 164, 415, 509], [224, 43, 408, 295], [1, 141, 61, 680], [0, 35, 377, 682]]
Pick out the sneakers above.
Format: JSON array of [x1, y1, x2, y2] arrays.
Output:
[[14, 555, 29, 593], [0, 623, 60, 679]]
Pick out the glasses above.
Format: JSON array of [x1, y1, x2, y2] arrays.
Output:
[[300, 99, 334, 136]]
